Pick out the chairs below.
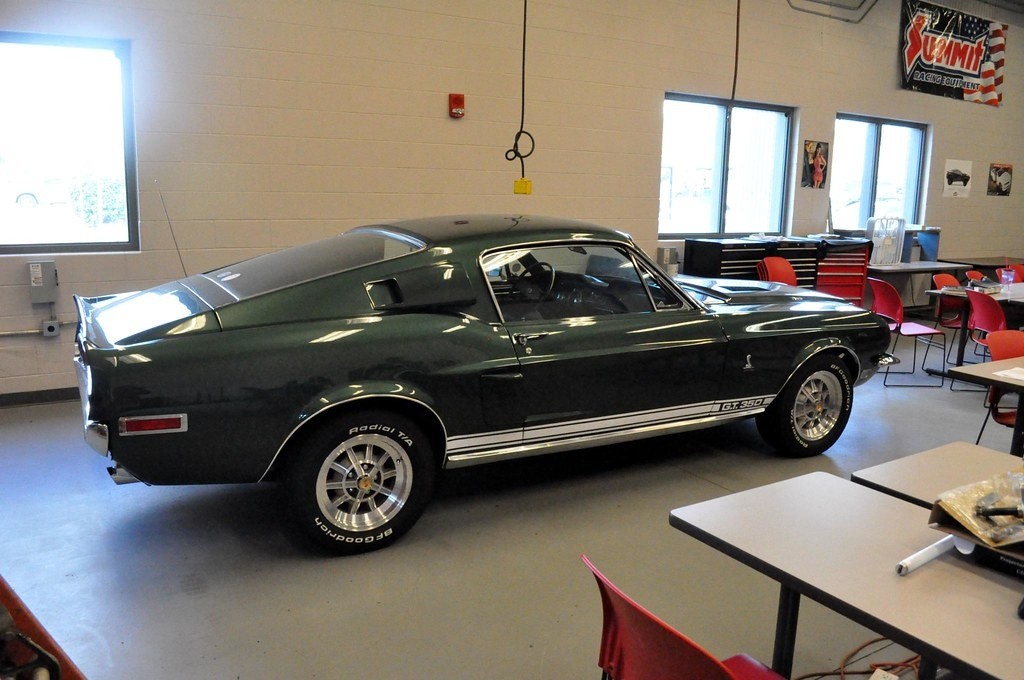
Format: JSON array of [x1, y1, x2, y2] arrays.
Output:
[[578, 255, 1024, 680]]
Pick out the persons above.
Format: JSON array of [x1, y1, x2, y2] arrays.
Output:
[[813, 143, 826, 188]]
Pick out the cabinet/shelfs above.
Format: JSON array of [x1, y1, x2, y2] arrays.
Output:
[[683, 234, 816, 307], [808, 235, 872, 309]]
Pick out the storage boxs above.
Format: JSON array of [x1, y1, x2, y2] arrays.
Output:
[[901, 227, 942, 263]]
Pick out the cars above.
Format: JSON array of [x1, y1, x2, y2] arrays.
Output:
[[72, 213, 900, 559]]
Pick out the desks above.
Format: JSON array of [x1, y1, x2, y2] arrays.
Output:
[[850, 440, 1024, 537], [667, 472, 1024, 680], [925, 283, 1024, 377], [867, 260, 973, 319], [937, 257, 1024, 268], [948, 355, 1024, 444]]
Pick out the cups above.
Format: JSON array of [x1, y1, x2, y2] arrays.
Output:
[[1001, 269, 1014, 292]]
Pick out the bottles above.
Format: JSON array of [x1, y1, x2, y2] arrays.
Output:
[[911, 238, 921, 263]]
